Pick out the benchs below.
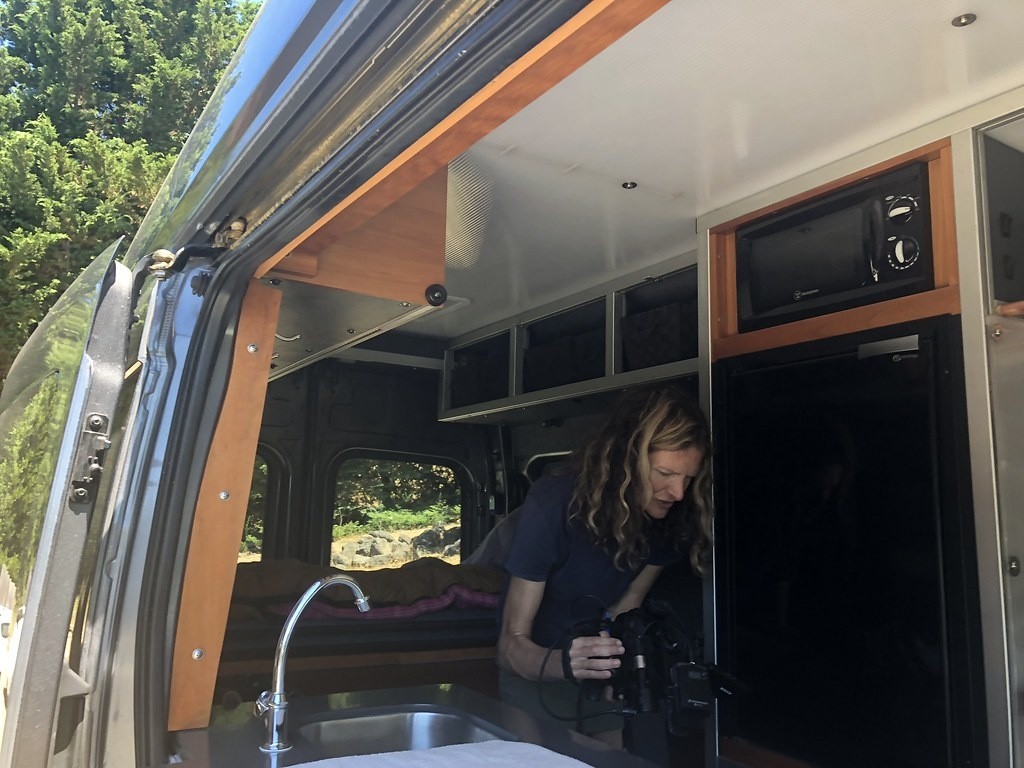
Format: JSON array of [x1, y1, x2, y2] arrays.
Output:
[[224, 593, 508, 658]]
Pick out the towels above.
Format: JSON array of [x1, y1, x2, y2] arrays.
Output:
[[275, 739, 597, 768]]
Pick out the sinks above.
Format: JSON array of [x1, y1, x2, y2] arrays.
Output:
[[288, 702, 511, 755]]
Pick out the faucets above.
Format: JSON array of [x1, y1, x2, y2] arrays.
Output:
[[252, 573, 372, 753]]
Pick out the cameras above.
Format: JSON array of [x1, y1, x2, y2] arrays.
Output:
[[595, 610, 672, 716]]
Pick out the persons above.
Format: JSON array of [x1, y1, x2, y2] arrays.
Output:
[[464, 382, 717, 749]]
